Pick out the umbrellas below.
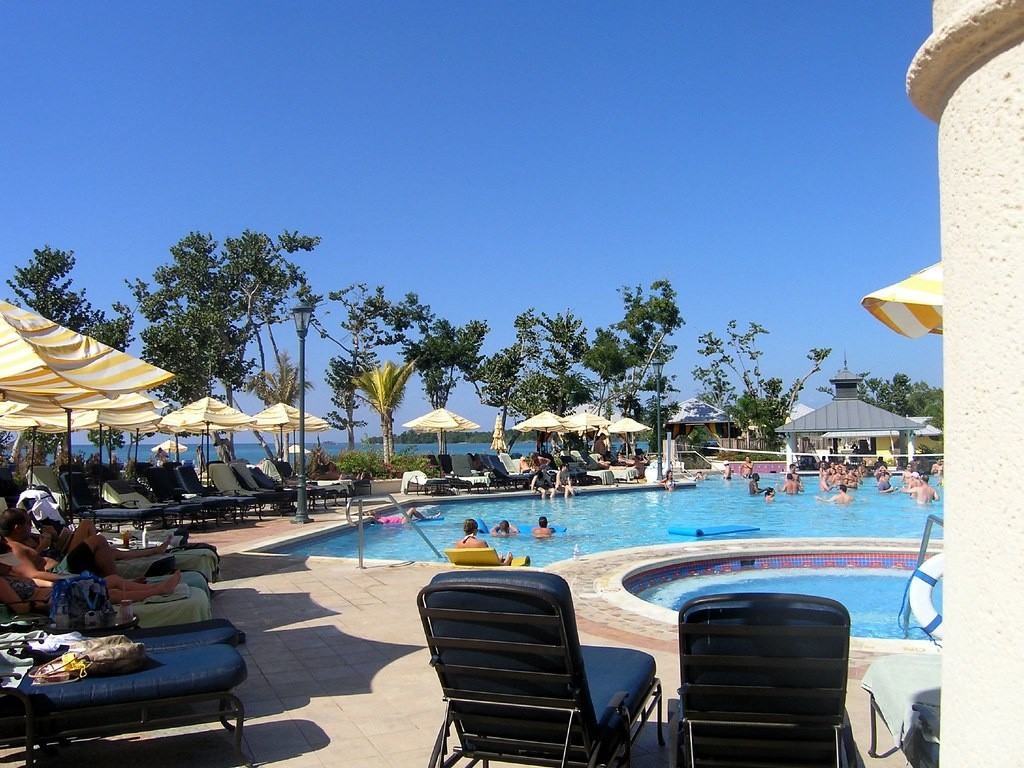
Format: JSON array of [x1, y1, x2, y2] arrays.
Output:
[[491, 413, 507, 454], [0, 298, 330, 524], [511, 411, 653, 459], [401, 407, 481, 455], [861, 260, 943, 339]]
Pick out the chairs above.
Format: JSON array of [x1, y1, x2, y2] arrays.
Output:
[[0, 450, 939, 768]]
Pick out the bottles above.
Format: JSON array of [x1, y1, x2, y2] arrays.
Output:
[[119, 600, 134, 630], [142, 528, 149, 548], [573, 545, 580, 560], [55, 593, 68, 628]]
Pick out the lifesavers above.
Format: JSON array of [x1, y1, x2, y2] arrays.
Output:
[[909, 552, 943, 638]]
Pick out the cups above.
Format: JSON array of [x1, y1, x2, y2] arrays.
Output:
[[122, 533, 129, 548]]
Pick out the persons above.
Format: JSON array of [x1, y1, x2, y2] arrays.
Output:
[[532, 517, 553, 535], [364, 507, 441, 524], [696, 472, 707, 479], [490, 521, 520, 535], [467, 433, 676, 499], [0, 507, 181, 616], [455, 519, 513, 566], [723, 460, 732, 480], [739, 443, 944, 504]]
